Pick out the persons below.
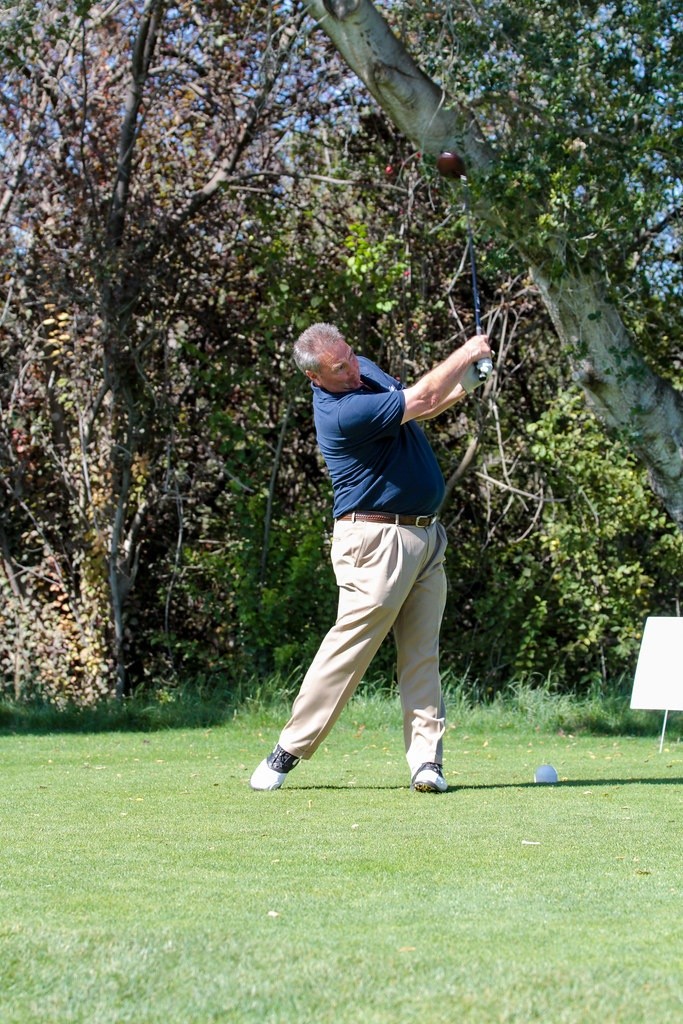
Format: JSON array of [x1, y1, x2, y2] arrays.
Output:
[[249, 324, 495, 792]]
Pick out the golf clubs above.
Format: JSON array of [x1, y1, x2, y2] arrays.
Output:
[[435, 150, 488, 382]]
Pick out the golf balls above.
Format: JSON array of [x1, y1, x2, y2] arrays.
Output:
[[533, 764, 558, 783]]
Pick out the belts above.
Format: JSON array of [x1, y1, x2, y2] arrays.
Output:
[[336, 510, 438, 528]]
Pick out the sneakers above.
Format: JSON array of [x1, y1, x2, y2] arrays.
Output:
[[249, 743, 300, 790], [412, 761, 448, 792]]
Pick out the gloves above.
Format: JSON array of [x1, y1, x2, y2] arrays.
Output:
[[459, 350, 495, 394]]
